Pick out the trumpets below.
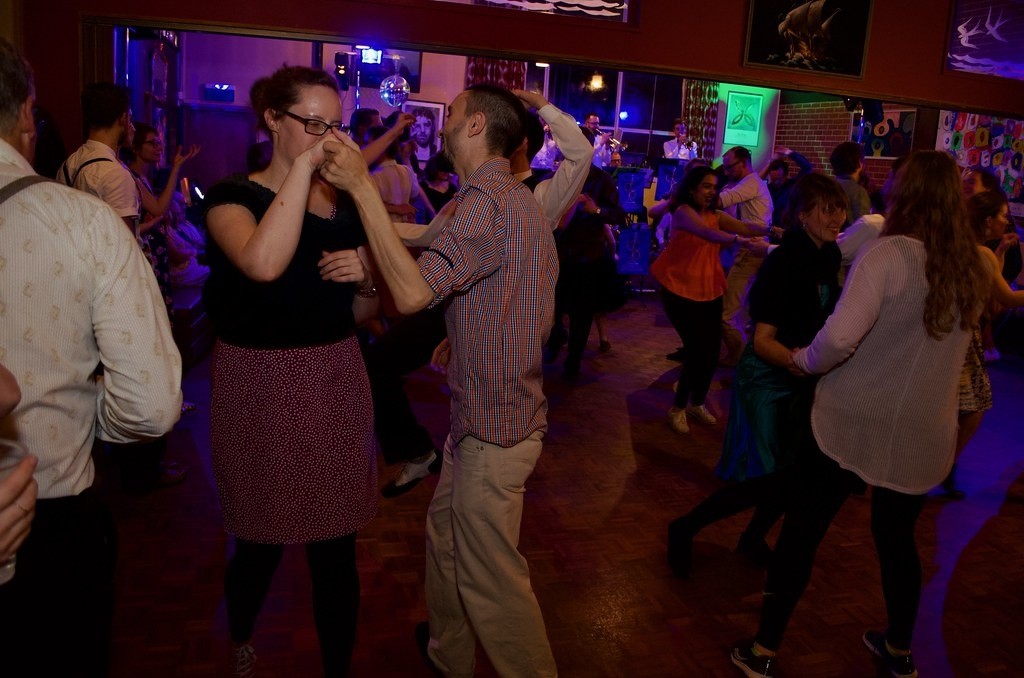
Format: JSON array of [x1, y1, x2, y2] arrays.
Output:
[[595, 127, 629, 152], [679, 132, 693, 151]]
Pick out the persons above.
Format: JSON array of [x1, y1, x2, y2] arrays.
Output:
[[584, 113, 613, 168], [202, 65, 379, 678], [649, 166, 787, 432], [529, 110, 557, 169], [320, 82, 564, 678], [344, 107, 459, 499], [748, 140, 910, 291], [939, 191, 1023, 501], [960, 169, 1000, 199], [0, 364, 37, 564], [651, 145, 816, 372], [663, 118, 698, 159], [54, 79, 199, 495], [724, 151, 994, 678], [411, 107, 438, 161], [507, 113, 629, 388], [0, 35, 183, 678], [664, 174, 847, 579]]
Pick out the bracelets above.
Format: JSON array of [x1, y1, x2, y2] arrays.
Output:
[[593, 207, 601, 214], [766, 222, 773, 233], [358, 266, 377, 296], [733, 234, 741, 243]]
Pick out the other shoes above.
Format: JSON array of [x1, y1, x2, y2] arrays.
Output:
[[158, 463, 187, 488], [730, 639, 777, 678], [717, 358, 737, 367], [686, 402, 716, 424], [543, 327, 568, 362], [228, 632, 258, 678], [561, 351, 581, 383], [667, 348, 683, 361], [110, 482, 143, 517], [667, 404, 689, 434], [181, 401, 196, 414], [381, 447, 443, 499], [735, 534, 774, 569], [942, 484, 965, 500], [862, 631, 917, 678], [667, 521, 693, 579], [599, 339, 611, 352], [415, 621, 445, 678]]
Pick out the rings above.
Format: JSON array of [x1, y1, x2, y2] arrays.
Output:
[[14, 500, 29, 514]]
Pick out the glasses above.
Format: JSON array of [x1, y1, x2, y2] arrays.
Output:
[[723, 160, 741, 172], [141, 138, 162, 148], [272, 105, 352, 135]]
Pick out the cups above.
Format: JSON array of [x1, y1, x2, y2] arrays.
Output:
[[0, 436, 30, 585]]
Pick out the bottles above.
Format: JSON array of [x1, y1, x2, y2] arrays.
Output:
[[637, 159, 652, 169]]
